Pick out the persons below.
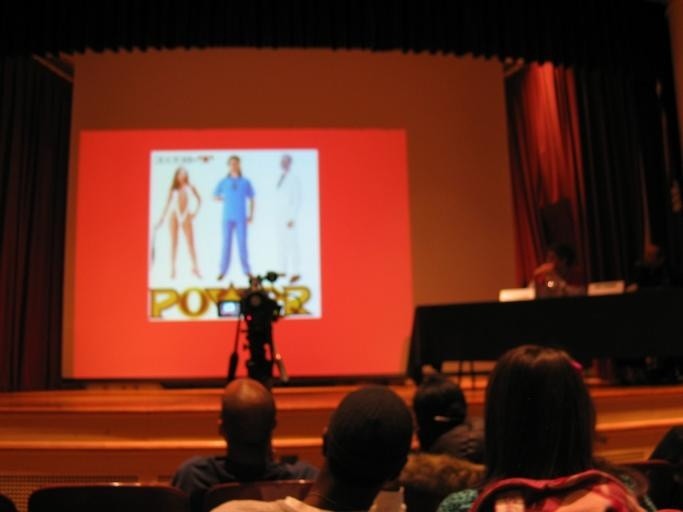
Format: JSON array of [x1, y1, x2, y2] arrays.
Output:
[[173, 378, 316, 489], [530, 241, 575, 300], [213, 156, 255, 281], [213, 386, 414, 511], [272, 156, 301, 283], [414, 376, 484, 464], [156, 169, 203, 280], [624, 244, 666, 293], [436, 344, 655, 511]]
